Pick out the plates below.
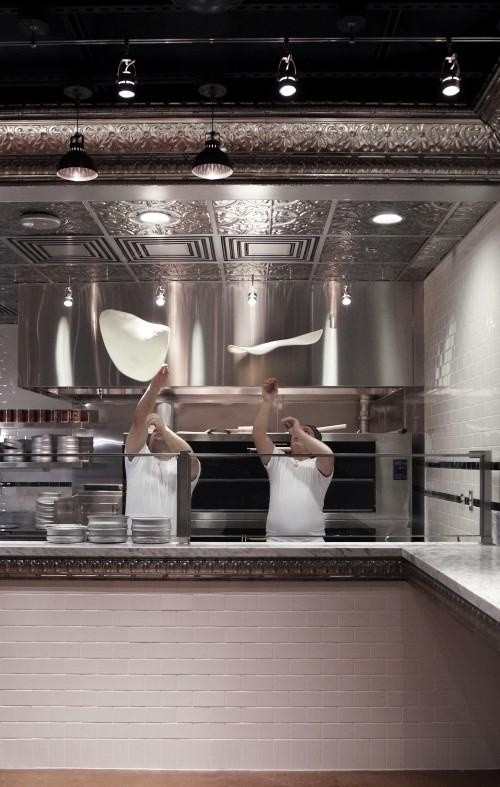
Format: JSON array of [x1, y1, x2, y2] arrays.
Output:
[[1, 409, 88, 422]]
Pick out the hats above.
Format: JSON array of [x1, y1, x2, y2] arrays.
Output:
[[306, 423, 323, 442]]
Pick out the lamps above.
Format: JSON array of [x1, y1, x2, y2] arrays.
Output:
[[191, 87, 237, 181], [340, 273, 353, 306], [152, 273, 167, 306], [109, 35, 471, 102], [247, 273, 258, 306], [62, 273, 75, 308], [54, 88, 101, 185]]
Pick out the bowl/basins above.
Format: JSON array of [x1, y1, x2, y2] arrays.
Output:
[[29, 433, 95, 463], [45, 515, 170, 544], [0, 439, 26, 463], [33, 490, 60, 528]]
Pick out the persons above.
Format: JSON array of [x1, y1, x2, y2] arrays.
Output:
[[122, 364, 204, 535], [250, 375, 337, 544]]
